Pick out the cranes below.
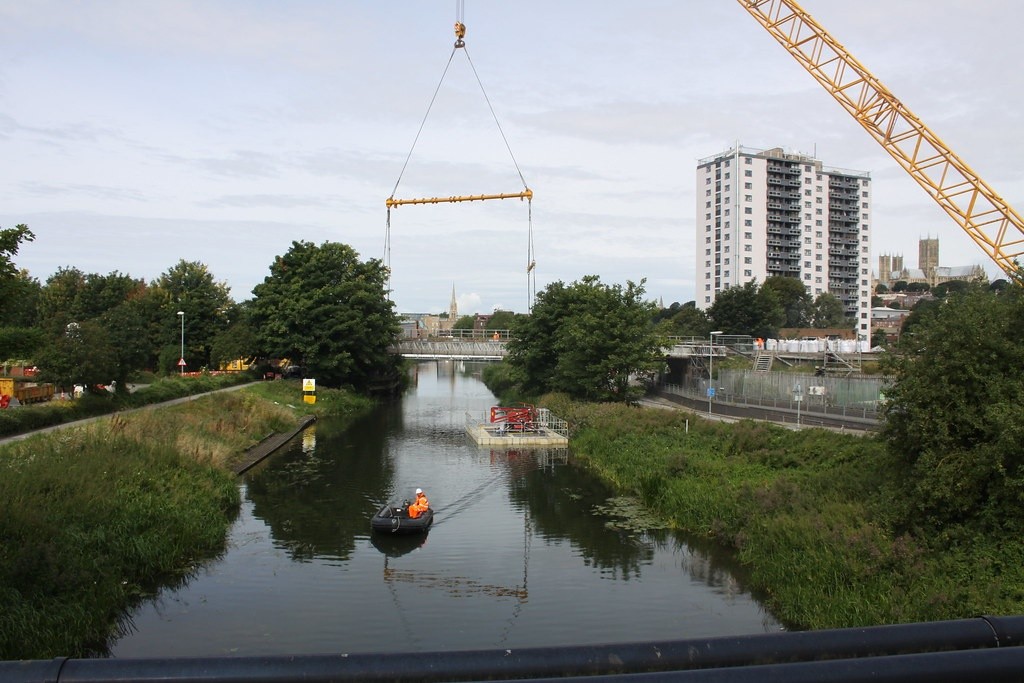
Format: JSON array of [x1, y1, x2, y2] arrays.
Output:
[[382, 0, 1024, 314]]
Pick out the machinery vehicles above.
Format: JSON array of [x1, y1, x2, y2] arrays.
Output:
[[220, 355, 291, 372]]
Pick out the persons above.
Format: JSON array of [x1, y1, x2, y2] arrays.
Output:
[[408, 487, 429, 519], [493, 332, 499, 340]]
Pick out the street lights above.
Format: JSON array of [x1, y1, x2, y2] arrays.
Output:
[[709, 331, 723, 414], [177, 311, 185, 381]]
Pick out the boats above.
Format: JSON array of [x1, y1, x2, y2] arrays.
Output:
[[371, 499, 433, 536]]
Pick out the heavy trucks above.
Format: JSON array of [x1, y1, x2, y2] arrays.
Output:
[[0, 375, 56, 405]]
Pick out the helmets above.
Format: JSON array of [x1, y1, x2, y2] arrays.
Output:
[[416, 488, 422, 495]]
